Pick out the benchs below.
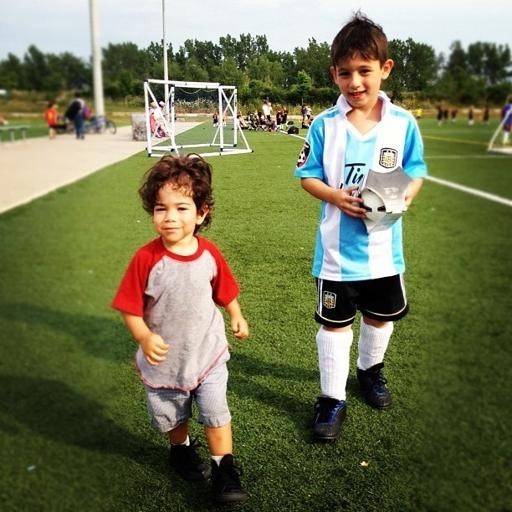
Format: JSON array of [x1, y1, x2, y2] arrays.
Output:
[[0, 124, 31, 142]]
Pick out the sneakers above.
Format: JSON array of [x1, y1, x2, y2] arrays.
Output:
[[356, 362, 392, 409], [210, 454, 245, 501], [313, 396, 346, 440], [168, 434, 211, 480]]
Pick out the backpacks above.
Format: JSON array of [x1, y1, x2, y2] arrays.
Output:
[[66, 101, 81, 120]]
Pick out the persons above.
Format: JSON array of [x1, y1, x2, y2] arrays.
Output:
[[149, 100, 165, 137], [407, 97, 512, 146], [68, 91, 86, 139], [43, 102, 59, 139], [112, 151, 251, 505], [294, 7, 428, 444], [213, 100, 314, 134]]
